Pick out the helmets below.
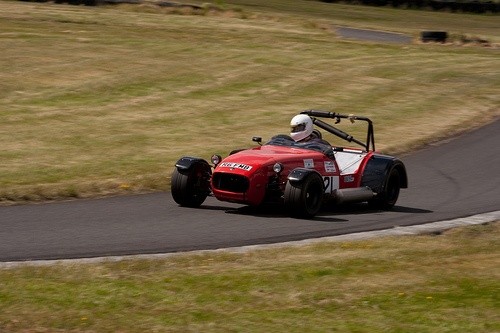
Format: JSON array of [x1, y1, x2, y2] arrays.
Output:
[[290, 113, 313, 142]]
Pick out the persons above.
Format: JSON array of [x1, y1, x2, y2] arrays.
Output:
[[289, 113, 319, 141]]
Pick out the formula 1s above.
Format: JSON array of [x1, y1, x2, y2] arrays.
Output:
[[171, 111, 408, 216]]
